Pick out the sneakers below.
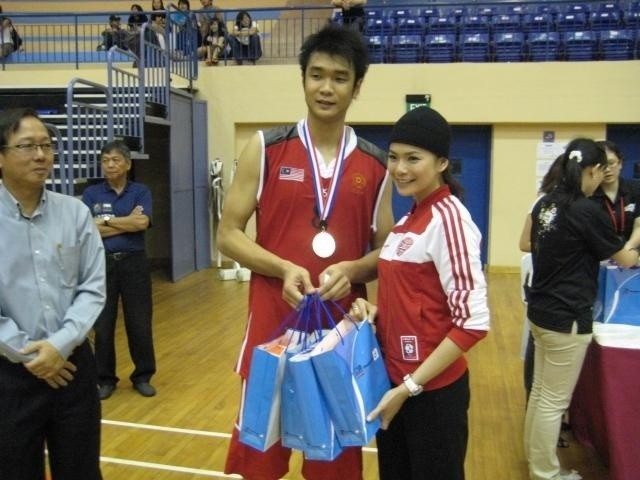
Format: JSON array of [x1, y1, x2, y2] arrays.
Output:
[[557, 436, 569, 447]]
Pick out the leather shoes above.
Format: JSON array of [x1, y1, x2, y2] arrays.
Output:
[[133, 382, 155, 396], [98, 384, 116, 399], [560, 467, 583, 480]]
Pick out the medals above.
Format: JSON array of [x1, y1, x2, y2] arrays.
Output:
[[310, 226, 337, 259]]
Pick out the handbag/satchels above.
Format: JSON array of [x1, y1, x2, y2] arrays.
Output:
[[288, 331, 346, 461], [311, 314, 392, 447], [603, 267, 640, 325], [280, 326, 306, 450], [238, 325, 311, 453], [592, 265, 605, 323]]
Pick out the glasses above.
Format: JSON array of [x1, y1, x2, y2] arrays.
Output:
[[0, 142, 56, 155], [607, 159, 621, 167]]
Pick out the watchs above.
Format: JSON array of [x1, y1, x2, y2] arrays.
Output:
[[103, 215, 111, 226], [403, 374, 424, 396]]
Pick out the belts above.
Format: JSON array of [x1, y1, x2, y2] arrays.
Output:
[[104, 251, 146, 261]]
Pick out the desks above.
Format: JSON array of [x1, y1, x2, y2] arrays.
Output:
[[525, 322, 640, 479]]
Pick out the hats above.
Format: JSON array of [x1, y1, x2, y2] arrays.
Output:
[[389, 106, 450, 159], [109, 13, 121, 21]]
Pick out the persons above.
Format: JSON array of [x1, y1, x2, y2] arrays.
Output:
[[215, 20, 396, 480], [519, 138, 639, 480], [348, 105, 492, 480], [81, 139, 158, 401], [96, 0, 265, 68], [331, 0, 367, 33], [0, 5, 25, 60], [1, 107, 108, 480]]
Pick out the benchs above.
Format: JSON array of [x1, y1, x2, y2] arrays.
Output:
[[1, 8, 280, 65]]
